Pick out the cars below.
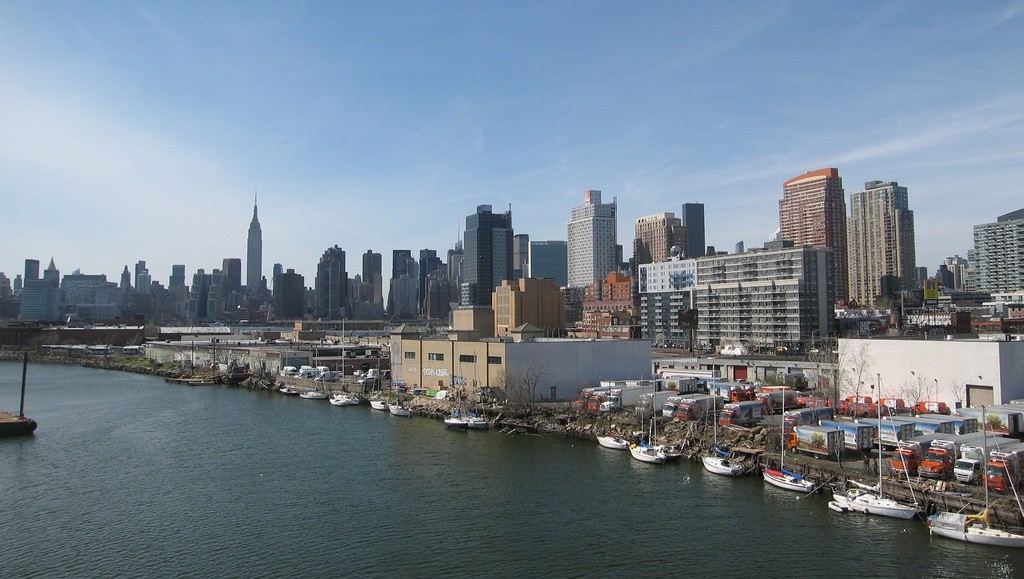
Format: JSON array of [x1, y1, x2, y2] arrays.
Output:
[[353, 370, 363, 376], [338, 371, 343, 377], [652, 342, 713, 350], [361, 370, 368, 378]]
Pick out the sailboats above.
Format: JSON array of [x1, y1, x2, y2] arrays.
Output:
[[762, 387, 818, 493], [388, 355, 414, 418], [926, 404, 1024, 549], [461, 349, 489, 431], [628, 374, 667, 464], [828, 372, 919, 520], [300, 347, 328, 399], [278, 353, 300, 396], [701, 361, 744, 477], [333, 318, 359, 405], [597, 380, 630, 450], [368, 358, 388, 411], [443, 357, 469, 432], [652, 375, 682, 462]]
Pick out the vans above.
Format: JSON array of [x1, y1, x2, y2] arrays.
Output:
[[380, 370, 390, 380], [367, 369, 379, 378]]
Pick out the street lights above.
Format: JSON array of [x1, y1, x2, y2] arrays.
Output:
[[812, 329, 820, 349]]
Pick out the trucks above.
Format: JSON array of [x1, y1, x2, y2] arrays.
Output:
[[779, 399, 1024, 494], [845, 396, 872, 404], [575, 386, 655, 412], [283, 366, 339, 382], [662, 382, 755, 421], [635, 391, 677, 414], [911, 402, 947, 417], [123, 347, 144, 354], [87, 347, 110, 355], [755, 392, 799, 414], [718, 401, 763, 427], [876, 399, 905, 413]]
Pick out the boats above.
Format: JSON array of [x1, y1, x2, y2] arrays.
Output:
[[329, 398, 347, 407], [189, 382, 216, 385]]
